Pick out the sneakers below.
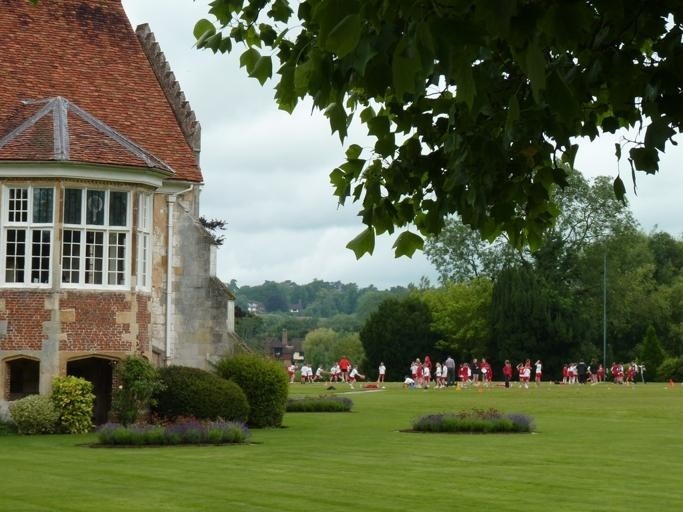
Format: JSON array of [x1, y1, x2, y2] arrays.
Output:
[[415, 381, 447, 389], [348, 384, 355, 389]]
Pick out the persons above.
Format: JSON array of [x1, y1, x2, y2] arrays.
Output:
[[558, 359, 647, 386], [522, 359, 532, 390], [287, 354, 365, 389], [515, 362, 525, 389], [403, 355, 493, 390], [534, 359, 542, 386], [502, 359, 512, 388], [376, 361, 386, 385]]
